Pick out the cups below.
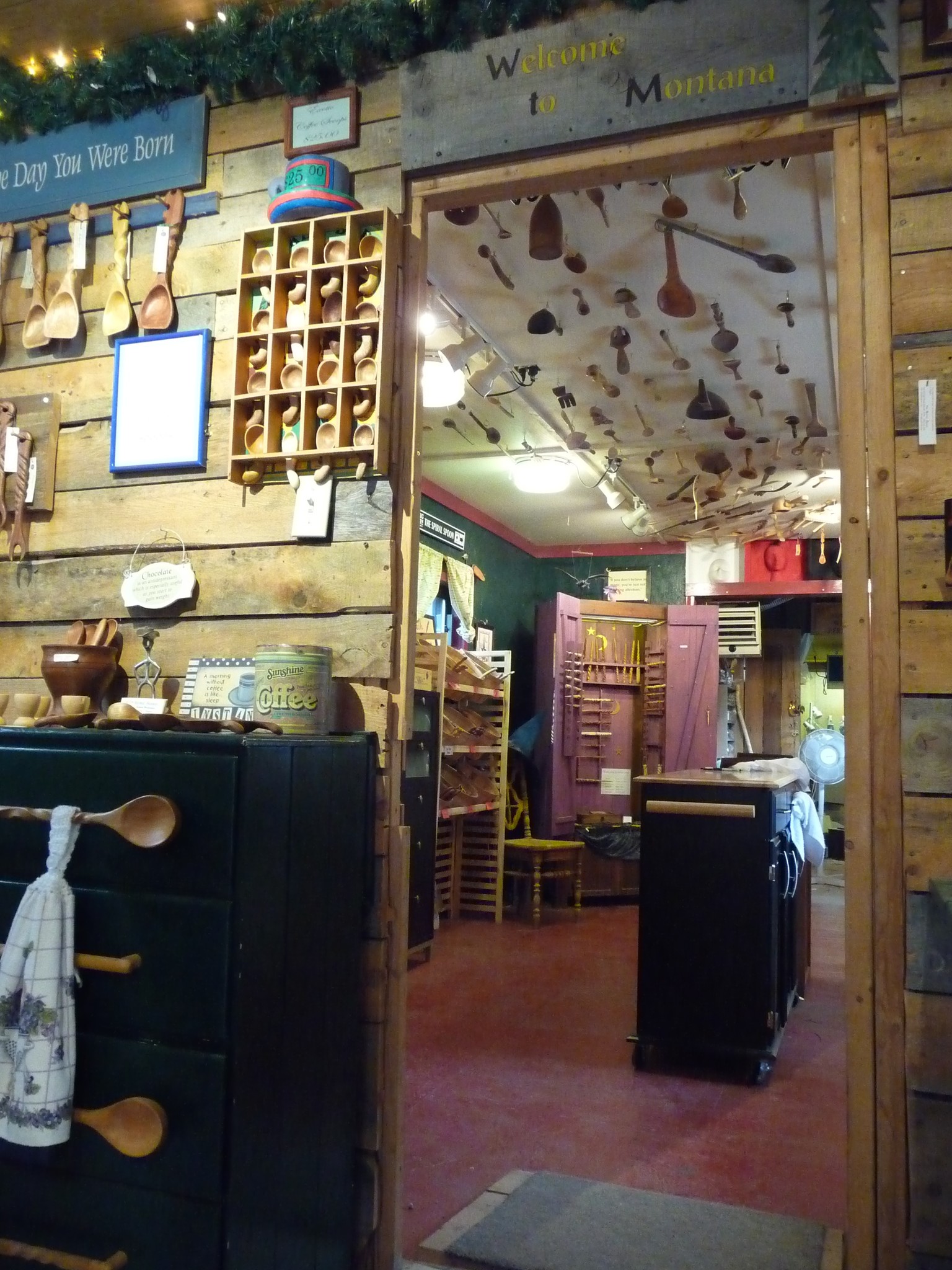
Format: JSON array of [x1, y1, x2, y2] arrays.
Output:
[[34, 696, 51, 720], [13, 693, 41, 727], [60, 695, 91, 715], [0, 694, 9, 726]]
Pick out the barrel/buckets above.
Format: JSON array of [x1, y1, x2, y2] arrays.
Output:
[[253, 643, 333, 736]]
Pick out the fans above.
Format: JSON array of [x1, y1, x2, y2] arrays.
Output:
[[799, 728, 845, 830]]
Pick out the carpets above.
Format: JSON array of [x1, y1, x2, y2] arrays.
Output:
[[447, 1173, 827, 1270]]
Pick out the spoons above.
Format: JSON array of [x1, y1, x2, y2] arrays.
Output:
[[101, 201, 133, 337], [42, 202, 89, 340], [242, 234, 383, 490], [65, 618, 118, 647], [106, 702, 284, 735], [0, 222, 15, 346], [139, 188, 186, 330], [22, 218, 52, 349]]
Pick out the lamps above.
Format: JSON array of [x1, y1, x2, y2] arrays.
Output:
[[422, 351, 466, 408], [466, 343, 507, 397], [438, 317, 485, 373], [511, 456, 573, 495], [599, 472, 626, 510], [632, 513, 651, 537], [621, 497, 647, 530], [804, 703, 823, 735]]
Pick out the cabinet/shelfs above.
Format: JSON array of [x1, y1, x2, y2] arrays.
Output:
[[0, 727, 379, 1270], [414, 629, 513, 924], [632, 769, 812, 1090], [399, 690, 440, 962], [535, 592, 719, 908], [227, 207, 401, 485], [716, 684, 737, 758]]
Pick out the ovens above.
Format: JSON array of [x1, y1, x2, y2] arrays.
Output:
[[0, 726, 377, 1270]]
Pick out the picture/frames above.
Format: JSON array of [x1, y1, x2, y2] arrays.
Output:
[[110, 328, 212, 473], [283, 86, 360, 158], [475, 623, 495, 663]]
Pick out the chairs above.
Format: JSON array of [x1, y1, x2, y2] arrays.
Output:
[[504, 755, 584, 927]]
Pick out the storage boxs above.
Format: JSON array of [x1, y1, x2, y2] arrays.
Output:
[[577, 812, 623, 824], [415, 667, 432, 691]]
